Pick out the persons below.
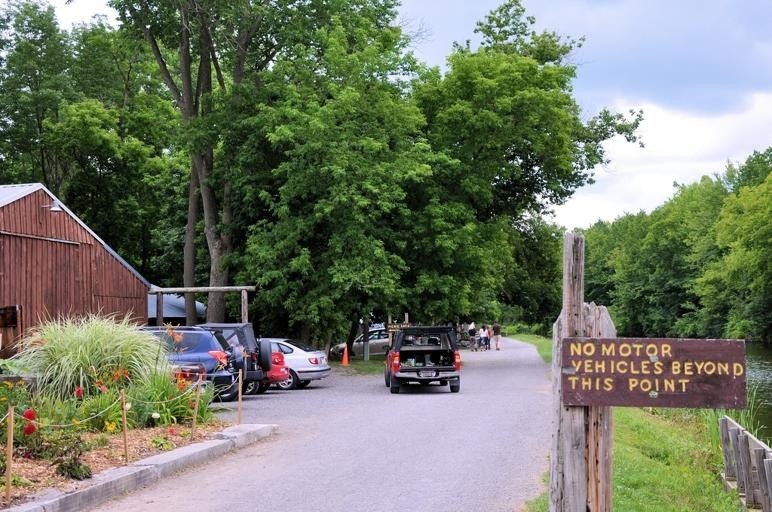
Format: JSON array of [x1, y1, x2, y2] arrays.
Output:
[[478, 325, 489, 351], [486, 326, 492, 351], [468, 329, 478, 352], [468, 321, 476, 330], [493, 320, 502, 351], [415, 336, 422, 346]]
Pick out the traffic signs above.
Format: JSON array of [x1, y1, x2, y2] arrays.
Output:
[[560, 336, 747, 409]]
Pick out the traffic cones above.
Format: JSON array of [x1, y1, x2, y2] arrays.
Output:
[[339, 344, 350, 366]]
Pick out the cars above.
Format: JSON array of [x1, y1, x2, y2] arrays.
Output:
[[330, 328, 416, 355], [129, 322, 334, 402]]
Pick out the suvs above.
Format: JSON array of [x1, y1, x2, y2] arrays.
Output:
[[383, 326, 461, 394]]
